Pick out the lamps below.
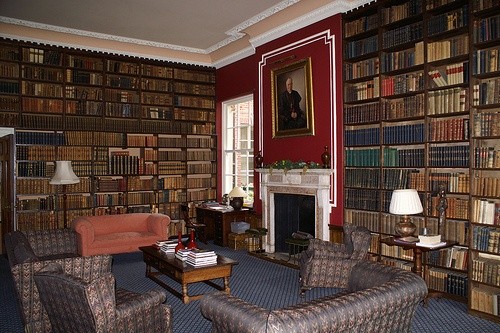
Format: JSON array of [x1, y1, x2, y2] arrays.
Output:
[[389, 189, 423, 238], [49, 161, 80, 229], [228, 187, 248, 211]]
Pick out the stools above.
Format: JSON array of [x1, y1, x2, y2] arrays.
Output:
[[228, 234, 246, 250], [284, 239, 309, 268]]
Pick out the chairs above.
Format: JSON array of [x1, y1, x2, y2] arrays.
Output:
[[182, 201, 207, 243]]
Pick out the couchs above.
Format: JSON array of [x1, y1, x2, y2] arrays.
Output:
[[199, 223, 428, 333], [4, 228, 113, 333], [69, 212, 170, 257], [33, 261, 173, 333]]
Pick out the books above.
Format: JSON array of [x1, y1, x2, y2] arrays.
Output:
[[0, 42, 218, 237], [155, 239, 217, 268], [342, 0, 500, 316]]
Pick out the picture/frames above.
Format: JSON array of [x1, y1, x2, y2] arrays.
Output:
[[270, 56, 315, 139]]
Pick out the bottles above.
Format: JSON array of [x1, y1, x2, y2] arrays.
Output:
[[175, 228, 197, 254]]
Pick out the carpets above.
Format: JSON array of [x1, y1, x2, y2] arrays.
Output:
[[114, 245, 359, 333]]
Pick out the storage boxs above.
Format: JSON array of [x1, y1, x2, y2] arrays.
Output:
[[231, 221, 250, 234]]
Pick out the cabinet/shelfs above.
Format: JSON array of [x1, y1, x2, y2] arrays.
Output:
[[0, 37, 249, 244], [343, 0, 500, 319]]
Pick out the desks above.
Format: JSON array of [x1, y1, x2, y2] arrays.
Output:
[[137, 246, 239, 305], [377, 236, 459, 307]]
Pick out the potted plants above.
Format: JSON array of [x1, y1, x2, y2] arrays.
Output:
[[244, 228, 268, 251]]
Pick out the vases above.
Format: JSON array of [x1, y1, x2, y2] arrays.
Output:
[[256, 151, 263, 168], [321, 145, 331, 168]]
